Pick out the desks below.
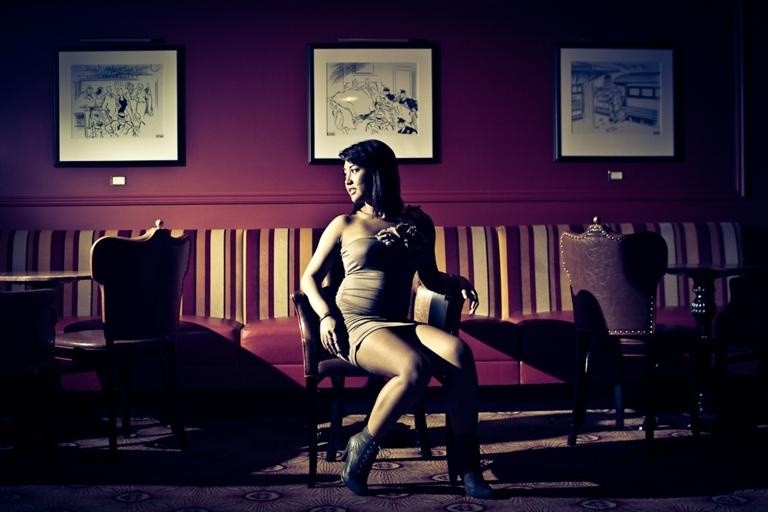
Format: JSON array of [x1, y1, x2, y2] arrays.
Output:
[[2, 272, 93, 437], [660, 261, 744, 438]]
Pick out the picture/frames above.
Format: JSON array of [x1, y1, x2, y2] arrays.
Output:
[[306, 41, 442, 166], [50, 40, 187, 169], [552, 45, 677, 162]]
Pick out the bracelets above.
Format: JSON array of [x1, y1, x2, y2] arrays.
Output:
[[319, 312, 333, 320]]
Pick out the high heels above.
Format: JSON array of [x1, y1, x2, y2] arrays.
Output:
[[448, 435, 493, 499], [339, 427, 378, 497]]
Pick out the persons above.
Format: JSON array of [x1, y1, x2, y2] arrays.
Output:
[[300, 140, 497, 499]]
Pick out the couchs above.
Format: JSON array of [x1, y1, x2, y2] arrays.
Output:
[[0, 223, 763, 391]]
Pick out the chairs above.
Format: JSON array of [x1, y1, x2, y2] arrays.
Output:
[[290, 284, 465, 487], [558, 215, 697, 447], [50, 228, 192, 459]]
